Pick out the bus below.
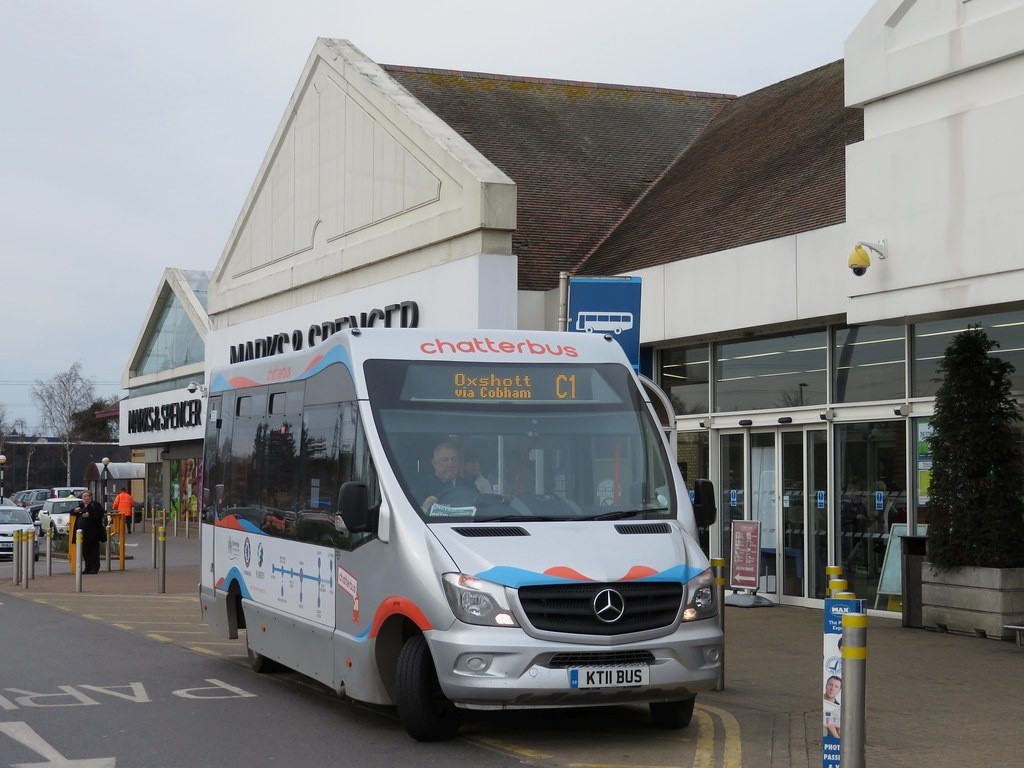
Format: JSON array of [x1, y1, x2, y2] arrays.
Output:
[[196, 327, 724, 744]]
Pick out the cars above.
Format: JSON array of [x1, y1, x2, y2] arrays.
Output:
[[0, 506, 42, 562], [0, 487, 52, 520], [35, 498, 85, 540]]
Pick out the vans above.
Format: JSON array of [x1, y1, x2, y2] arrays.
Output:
[[51, 487, 88, 499]]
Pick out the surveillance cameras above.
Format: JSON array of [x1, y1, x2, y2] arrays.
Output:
[[187, 381, 207, 398], [848, 240, 887, 275]]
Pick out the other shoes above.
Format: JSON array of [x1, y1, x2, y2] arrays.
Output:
[[82, 568, 98, 574]]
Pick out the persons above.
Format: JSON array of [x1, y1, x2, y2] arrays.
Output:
[[417, 441, 484, 512], [70, 491, 106, 574], [843, 481, 892, 566], [111, 488, 134, 535]]
